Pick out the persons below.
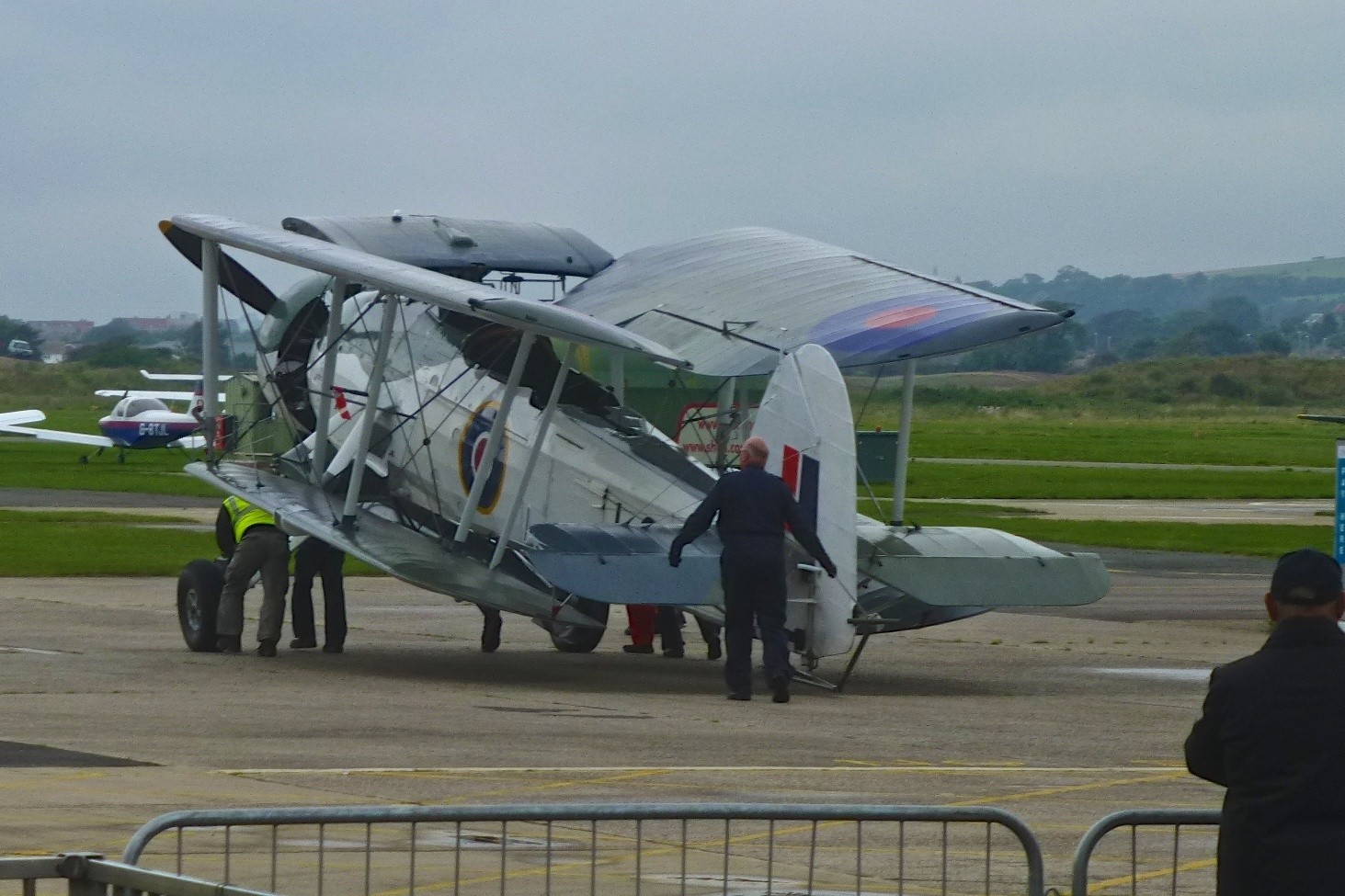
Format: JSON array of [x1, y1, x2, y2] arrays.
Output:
[[288, 529, 350, 652], [653, 601, 725, 660], [666, 437, 839, 702], [215, 496, 292, 655], [621, 602, 688, 655], [1185, 548, 1345, 896]]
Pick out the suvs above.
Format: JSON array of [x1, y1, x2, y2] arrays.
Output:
[[7, 340, 33, 358]]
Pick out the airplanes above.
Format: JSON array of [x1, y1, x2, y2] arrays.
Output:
[[0, 369, 235, 465], [157, 212, 1112, 697]]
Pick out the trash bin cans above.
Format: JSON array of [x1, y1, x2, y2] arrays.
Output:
[[856, 431, 899, 485]]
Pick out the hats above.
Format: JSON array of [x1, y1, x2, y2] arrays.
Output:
[[1271, 549, 1343, 604]]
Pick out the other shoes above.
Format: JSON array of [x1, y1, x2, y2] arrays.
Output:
[[216, 635, 242, 652], [322, 641, 343, 653], [708, 637, 722, 660], [257, 639, 277, 656], [290, 637, 317, 648], [663, 646, 685, 658], [773, 673, 790, 703], [623, 643, 655, 653], [481, 614, 502, 652], [726, 690, 751, 700]]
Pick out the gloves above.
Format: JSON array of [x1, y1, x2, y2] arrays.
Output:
[[668, 541, 683, 567], [824, 561, 836, 579]]
